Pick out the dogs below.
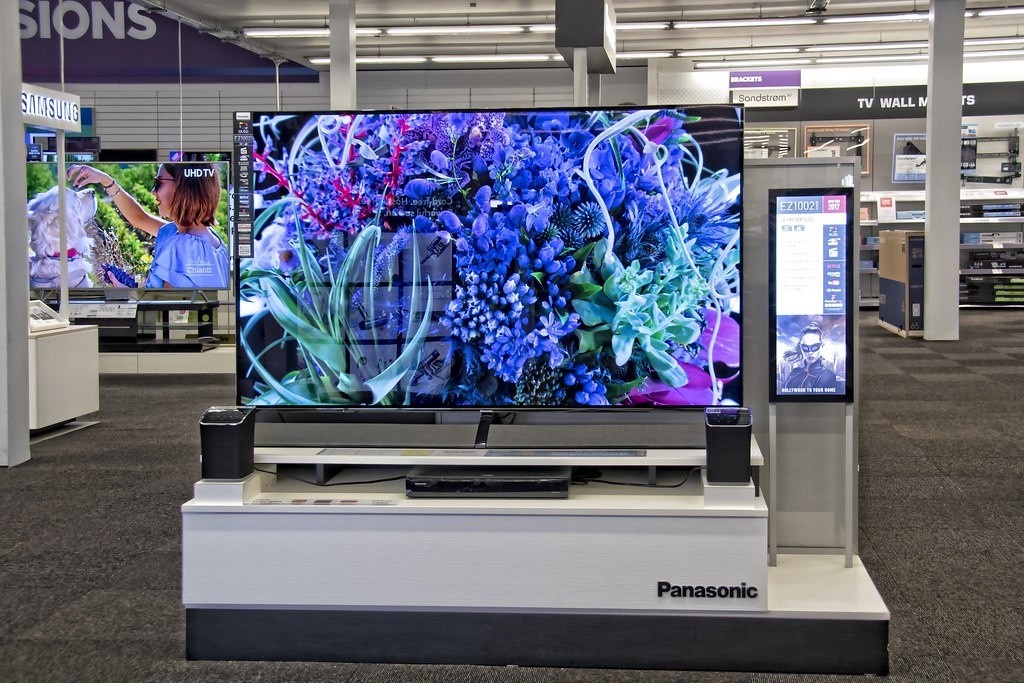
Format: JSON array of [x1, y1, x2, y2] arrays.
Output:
[[26, 185, 97, 289]]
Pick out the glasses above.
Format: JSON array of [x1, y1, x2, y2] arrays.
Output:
[[799, 342, 822, 352], [153, 177, 180, 192]]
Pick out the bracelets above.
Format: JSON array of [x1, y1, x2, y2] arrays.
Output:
[[102, 179, 121, 198]]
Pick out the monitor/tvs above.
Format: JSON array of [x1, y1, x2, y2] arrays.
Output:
[[230, 102, 744, 457], [25, 148, 229, 303]]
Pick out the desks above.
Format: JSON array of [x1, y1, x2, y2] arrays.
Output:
[[29, 324, 100, 429]]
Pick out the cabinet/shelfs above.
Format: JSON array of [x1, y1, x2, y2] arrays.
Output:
[[179, 434, 891, 677], [43, 299, 220, 352], [859, 219, 1024, 310]]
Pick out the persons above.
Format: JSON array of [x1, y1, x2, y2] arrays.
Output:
[[783, 323, 836, 392], [67, 164, 228, 289]]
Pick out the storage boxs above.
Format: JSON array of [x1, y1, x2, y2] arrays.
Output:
[[878, 230, 924, 339]]
